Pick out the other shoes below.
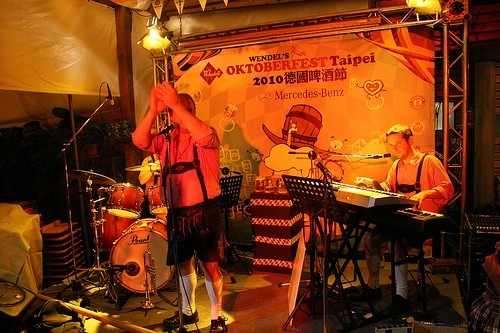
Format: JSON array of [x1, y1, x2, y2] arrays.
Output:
[[209, 316, 228, 333], [350, 285, 382, 301], [381, 295, 412, 316], [163, 310, 199, 325]]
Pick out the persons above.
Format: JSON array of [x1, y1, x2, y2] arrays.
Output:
[[132, 82, 227, 333], [346, 124, 455, 318]]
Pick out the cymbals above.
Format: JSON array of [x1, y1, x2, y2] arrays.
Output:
[[66, 170, 116, 186], [125, 163, 161, 172]]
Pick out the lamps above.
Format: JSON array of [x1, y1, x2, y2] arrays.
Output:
[[445, 0, 468, 21], [146, 16, 162, 33]]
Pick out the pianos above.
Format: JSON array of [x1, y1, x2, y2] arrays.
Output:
[[308, 177, 444, 325]]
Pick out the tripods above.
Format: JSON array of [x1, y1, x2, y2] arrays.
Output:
[[126, 253, 167, 315], [282, 177, 349, 333], [86, 180, 111, 285], [56, 100, 106, 298], [217, 175, 245, 268]]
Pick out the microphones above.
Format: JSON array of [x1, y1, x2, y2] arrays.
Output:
[[367, 153, 391, 159], [156, 124, 173, 135], [314, 161, 328, 176], [106, 83, 114, 105]]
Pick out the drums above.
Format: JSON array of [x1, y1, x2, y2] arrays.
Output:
[[106, 184, 144, 218], [101, 208, 137, 251], [109, 218, 177, 294], [146, 186, 168, 214]]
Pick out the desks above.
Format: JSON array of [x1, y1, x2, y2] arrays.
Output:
[[249, 192, 304, 274]]
